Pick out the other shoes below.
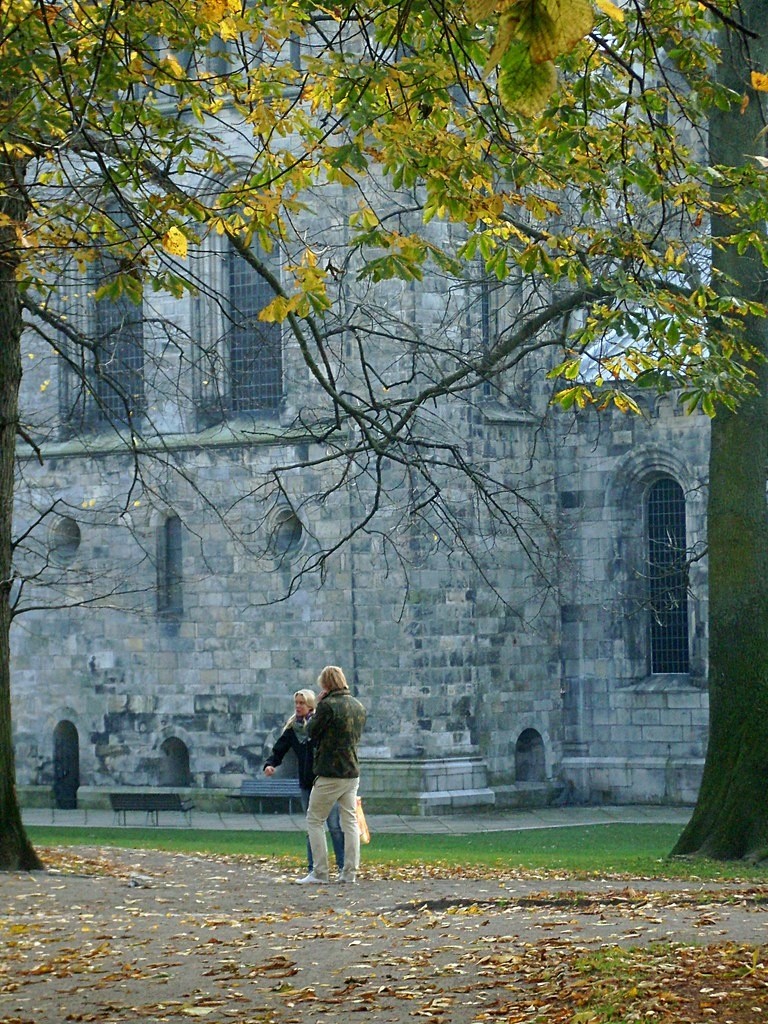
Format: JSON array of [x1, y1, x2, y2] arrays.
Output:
[[338, 875, 355, 882], [295, 875, 327, 884]]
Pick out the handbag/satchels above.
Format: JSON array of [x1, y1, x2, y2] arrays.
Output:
[[357, 796, 370, 845]]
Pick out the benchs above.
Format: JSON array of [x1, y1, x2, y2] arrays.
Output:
[[110, 792, 195, 827], [224, 778, 303, 815]]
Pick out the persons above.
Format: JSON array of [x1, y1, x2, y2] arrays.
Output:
[[295, 666, 368, 887], [260, 688, 345, 877]]
